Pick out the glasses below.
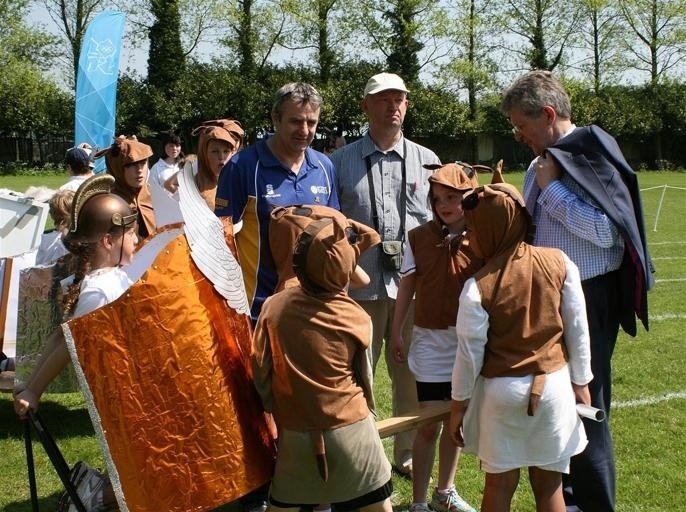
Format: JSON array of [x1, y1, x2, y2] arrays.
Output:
[[512, 113, 537, 134]]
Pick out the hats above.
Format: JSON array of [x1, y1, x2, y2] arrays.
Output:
[[64, 147, 89, 161], [364, 72, 411, 99]]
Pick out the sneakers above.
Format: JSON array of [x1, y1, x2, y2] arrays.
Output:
[[60, 461, 105, 512], [430, 487, 477, 512]]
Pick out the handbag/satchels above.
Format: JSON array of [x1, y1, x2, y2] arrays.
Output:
[[378, 240, 406, 271]]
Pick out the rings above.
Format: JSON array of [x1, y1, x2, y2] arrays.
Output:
[[536, 156, 544, 164]]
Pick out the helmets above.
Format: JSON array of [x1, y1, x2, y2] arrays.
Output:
[[64, 175, 139, 243]]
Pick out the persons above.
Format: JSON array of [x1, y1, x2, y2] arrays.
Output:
[[327, 71, 441, 481], [448, 184, 594, 510], [212, 84, 343, 416], [501, 71, 657, 507], [14, 176, 246, 511], [389, 161, 481, 511], [249, 203, 395, 511], [21, 119, 245, 266]]
